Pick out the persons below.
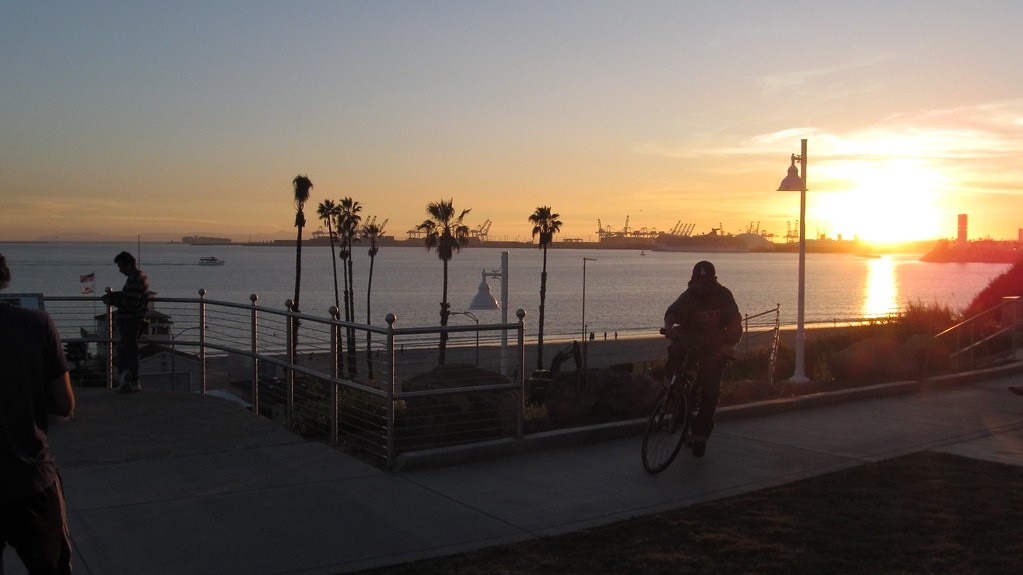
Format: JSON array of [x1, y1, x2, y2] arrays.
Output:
[[604, 332, 607, 339], [101, 251, 149, 393], [614, 331, 617, 339], [0, 252, 76, 575], [590, 332, 594, 339], [664, 260, 744, 458]]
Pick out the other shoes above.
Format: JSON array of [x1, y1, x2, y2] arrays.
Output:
[[120, 382, 142, 393], [692, 436, 705, 456]]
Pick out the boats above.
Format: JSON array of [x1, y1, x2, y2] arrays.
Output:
[[199, 256, 225, 266]]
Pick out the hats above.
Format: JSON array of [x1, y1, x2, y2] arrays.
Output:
[[693, 261, 715, 281]]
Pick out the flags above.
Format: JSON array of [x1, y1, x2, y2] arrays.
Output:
[[80, 273, 96, 283], [81, 285, 94, 293]]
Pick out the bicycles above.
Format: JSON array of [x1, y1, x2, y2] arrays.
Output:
[[642, 327, 739, 474]]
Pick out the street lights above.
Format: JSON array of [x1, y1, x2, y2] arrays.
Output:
[[172, 324, 210, 391], [450, 311, 479, 368], [468, 251, 508, 378], [581, 257, 596, 368], [776, 139, 811, 385]]
